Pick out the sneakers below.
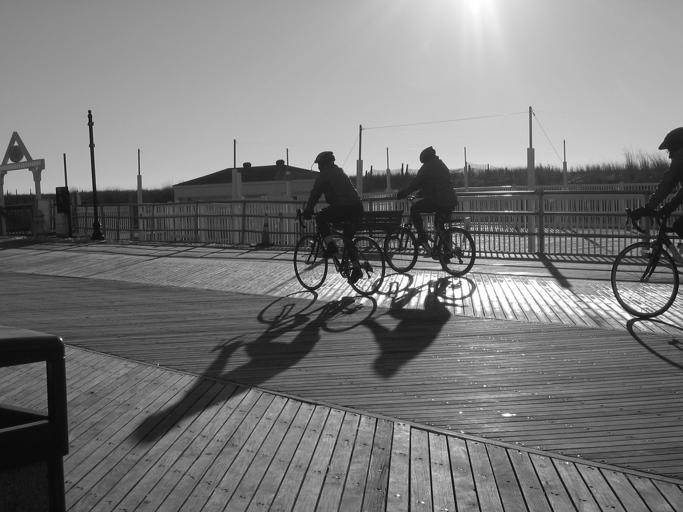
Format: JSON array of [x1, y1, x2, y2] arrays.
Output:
[[322, 242, 339, 258], [443, 247, 453, 258], [412, 236, 428, 245], [348, 267, 363, 285]]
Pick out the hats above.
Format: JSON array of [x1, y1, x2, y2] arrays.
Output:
[[659, 127, 683, 149]]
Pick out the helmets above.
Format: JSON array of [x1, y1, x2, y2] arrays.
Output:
[[420, 147, 435, 163], [314, 151, 335, 163]]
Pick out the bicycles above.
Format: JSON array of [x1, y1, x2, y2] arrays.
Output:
[[292, 206, 386, 298], [382, 188, 477, 278], [610, 201, 683, 319]]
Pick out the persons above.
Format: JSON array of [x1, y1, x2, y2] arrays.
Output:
[[629, 126, 683, 240], [396, 145, 459, 261], [303, 149, 363, 284]]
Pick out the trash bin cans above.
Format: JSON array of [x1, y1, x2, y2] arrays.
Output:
[[0, 325, 70, 512]]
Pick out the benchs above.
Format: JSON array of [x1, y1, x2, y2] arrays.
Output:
[[322, 209, 404, 260]]
